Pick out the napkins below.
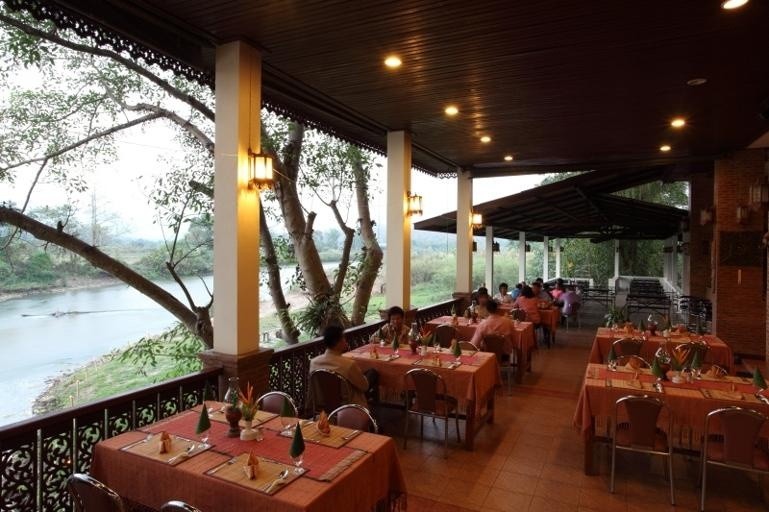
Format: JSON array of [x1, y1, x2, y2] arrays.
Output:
[[432, 354, 441, 366], [368, 343, 378, 357], [242, 448, 260, 480], [720, 380, 744, 400], [316, 411, 331, 436], [160, 431, 171, 453], [627, 371, 641, 389]]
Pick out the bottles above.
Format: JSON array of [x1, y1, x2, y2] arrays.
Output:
[[656, 340, 670, 365], [223, 376, 243, 408], [407, 323, 420, 340]]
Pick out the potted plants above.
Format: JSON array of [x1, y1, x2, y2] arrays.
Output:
[[225, 379, 241, 436]]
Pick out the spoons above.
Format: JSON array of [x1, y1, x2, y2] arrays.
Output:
[[261, 469, 289, 493]]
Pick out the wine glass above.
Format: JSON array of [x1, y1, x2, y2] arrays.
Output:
[[292, 454, 304, 473], [198, 430, 210, 449]]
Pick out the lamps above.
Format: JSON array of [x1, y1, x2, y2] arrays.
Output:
[[698, 204, 713, 227], [471, 211, 483, 226], [407, 191, 422, 215], [246, 146, 277, 189], [749, 175, 769, 216], [735, 199, 750, 226]]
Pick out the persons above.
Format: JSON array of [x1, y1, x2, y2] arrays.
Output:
[[309, 323, 378, 408], [470, 277, 584, 366], [371, 306, 411, 346]]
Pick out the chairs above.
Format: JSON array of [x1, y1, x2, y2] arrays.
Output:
[[308, 368, 376, 421], [253, 391, 301, 417], [400, 370, 460, 459], [160, 500, 199, 510], [585, 279, 709, 373], [699, 406, 769, 512], [328, 406, 379, 433], [67, 473, 126, 511], [415, 280, 584, 395], [609, 395, 675, 506]]
